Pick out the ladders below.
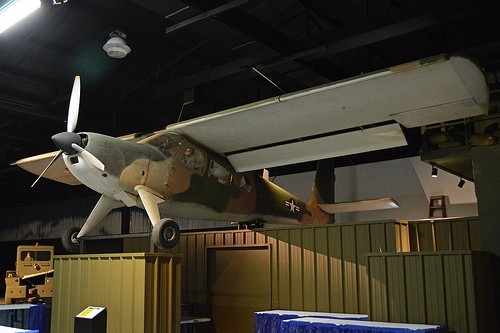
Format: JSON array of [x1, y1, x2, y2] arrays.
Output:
[[429, 196, 448, 218]]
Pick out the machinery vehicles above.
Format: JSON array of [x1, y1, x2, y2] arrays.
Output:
[[4, 244, 53, 305]]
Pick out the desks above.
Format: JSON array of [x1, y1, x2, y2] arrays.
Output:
[[0, 304, 37, 329], [254, 310, 445, 333]]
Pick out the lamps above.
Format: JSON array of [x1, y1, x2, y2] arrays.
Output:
[[431, 166, 438, 177], [103, 29, 132, 58], [458, 177, 465, 189]]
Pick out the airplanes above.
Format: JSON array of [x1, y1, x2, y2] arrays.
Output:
[[10, 54, 490, 251]]
